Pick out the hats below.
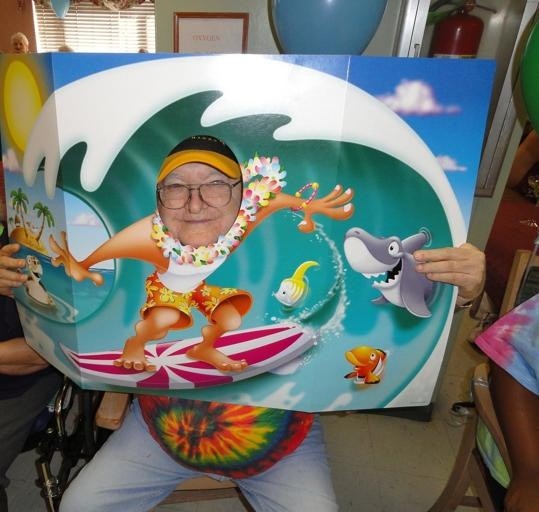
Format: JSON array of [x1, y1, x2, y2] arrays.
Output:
[[155, 133, 242, 184]]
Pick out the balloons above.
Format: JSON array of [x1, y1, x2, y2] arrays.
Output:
[[514, 17, 539, 141], [268, 2, 390, 55], [49, 0, 72, 21]]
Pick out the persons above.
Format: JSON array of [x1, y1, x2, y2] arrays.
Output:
[[468, 290, 539, 512], [0, 134, 489, 512], [0, 156, 70, 512], [10, 30, 30, 54]]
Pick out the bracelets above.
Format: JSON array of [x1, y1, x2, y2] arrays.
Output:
[[459, 295, 478, 309]]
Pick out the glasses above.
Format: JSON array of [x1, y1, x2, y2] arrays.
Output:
[[155, 179, 242, 209]]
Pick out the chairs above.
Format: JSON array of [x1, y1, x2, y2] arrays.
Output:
[[426, 250, 539, 512], [21, 365, 239, 512]]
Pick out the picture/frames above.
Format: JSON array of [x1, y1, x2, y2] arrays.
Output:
[[174, 12, 250, 56]]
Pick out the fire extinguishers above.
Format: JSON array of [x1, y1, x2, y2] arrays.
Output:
[[429, 0, 497, 59]]
